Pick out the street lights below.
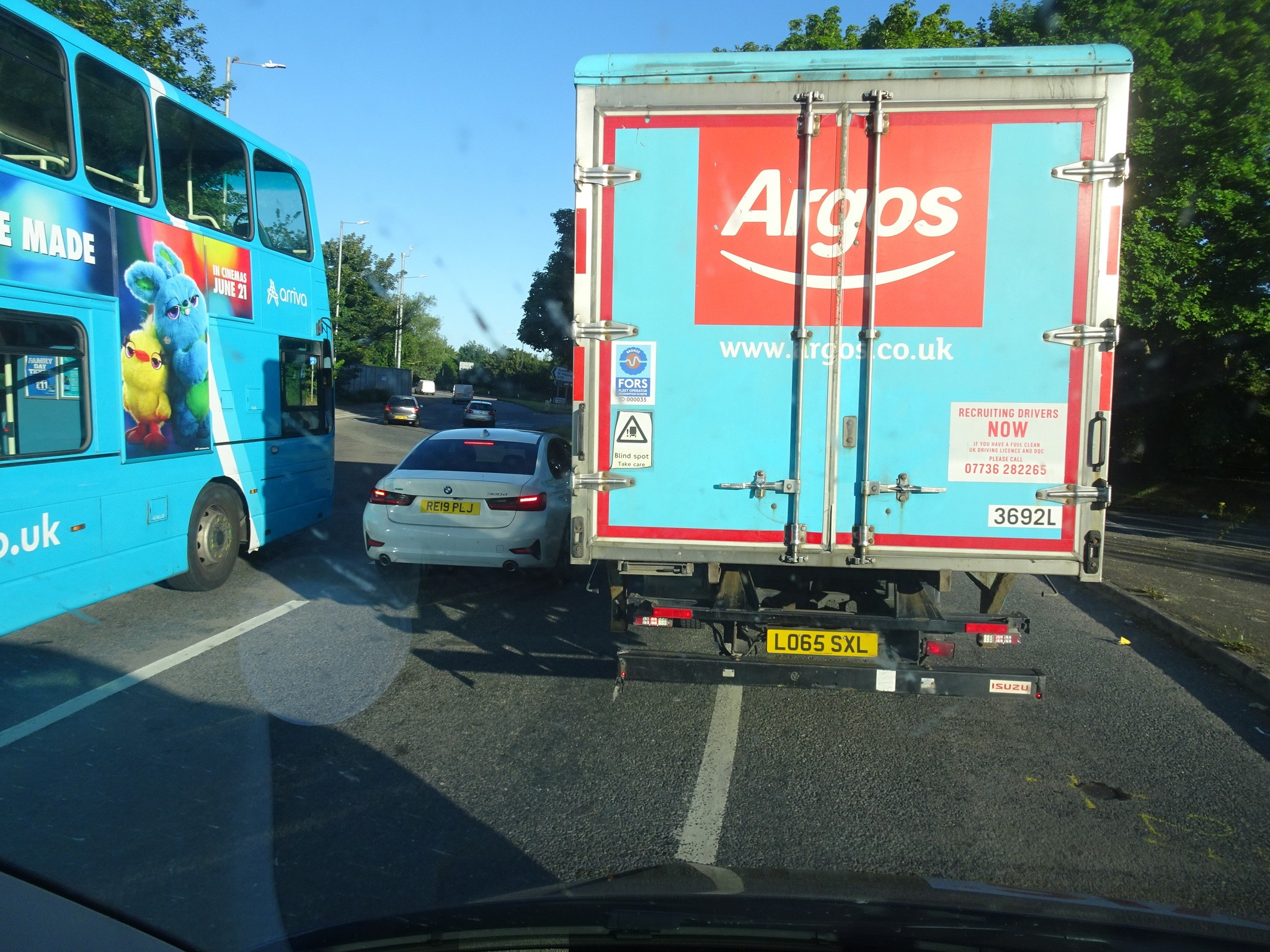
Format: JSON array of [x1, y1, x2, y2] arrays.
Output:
[[221, 55, 286, 229], [507, 331, 523, 400], [334, 220, 370, 365], [394, 245, 428, 368]]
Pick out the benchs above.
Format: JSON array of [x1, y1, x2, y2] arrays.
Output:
[[430, 450, 529, 472]]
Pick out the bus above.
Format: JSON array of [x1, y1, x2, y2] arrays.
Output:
[[0, 0, 336, 638]]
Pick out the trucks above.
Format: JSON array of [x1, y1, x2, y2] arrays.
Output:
[[570, 42, 1135, 700]]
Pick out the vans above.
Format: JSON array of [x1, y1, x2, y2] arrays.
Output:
[[452, 384, 473, 404], [414, 380, 435, 396]]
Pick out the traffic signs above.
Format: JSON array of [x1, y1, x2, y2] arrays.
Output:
[[551, 366, 573, 383], [554, 380, 570, 388], [553, 397, 566, 405]]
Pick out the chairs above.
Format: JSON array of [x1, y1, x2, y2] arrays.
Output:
[[194, 211, 274, 248]]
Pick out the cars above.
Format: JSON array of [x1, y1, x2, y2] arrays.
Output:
[[463, 401, 498, 428], [383, 395, 423, 428], [363, 428, 572, 591]]
